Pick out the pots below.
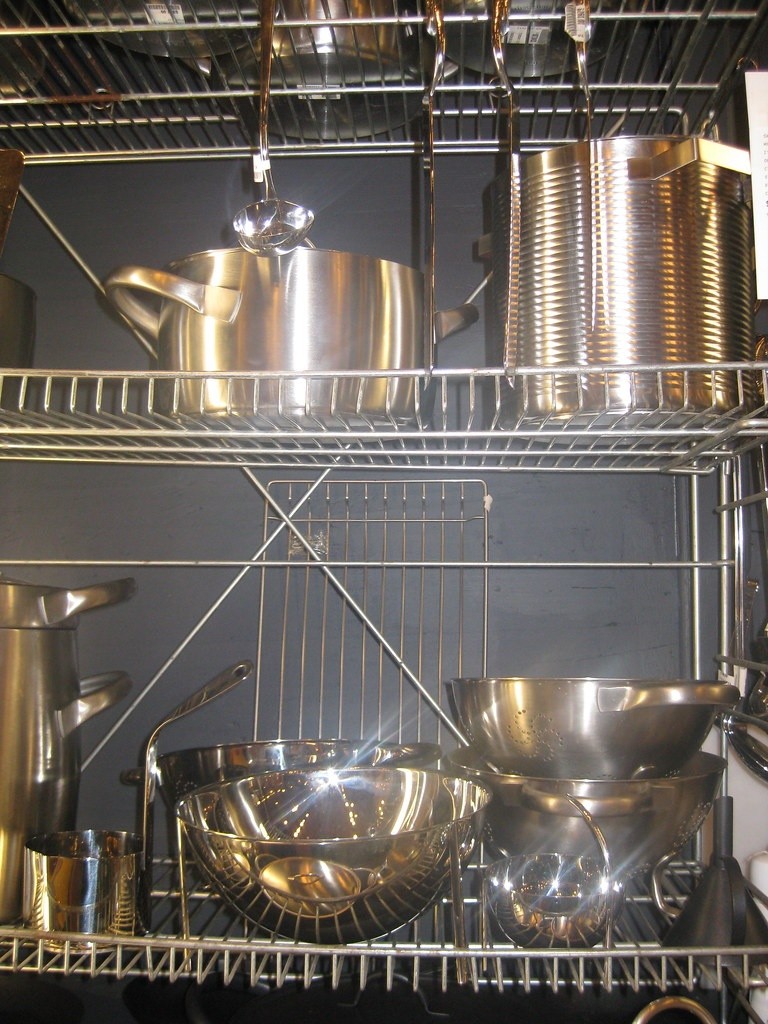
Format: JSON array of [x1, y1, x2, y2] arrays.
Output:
[[120, 736, 441, 805], [104, 248, 481, 443], [473, 131, 768, 445], [0, 0, 620, 140]]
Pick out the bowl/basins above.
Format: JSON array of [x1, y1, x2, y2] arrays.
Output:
[[442, 747, 730, 883], [173, 767, 494, 943], [448, 676, 740, 780]]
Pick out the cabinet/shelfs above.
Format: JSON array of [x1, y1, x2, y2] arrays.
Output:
[[2, 1, 768, 1024]]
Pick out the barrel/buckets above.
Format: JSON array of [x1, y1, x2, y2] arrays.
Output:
[[0, 574, 135, 926]]
